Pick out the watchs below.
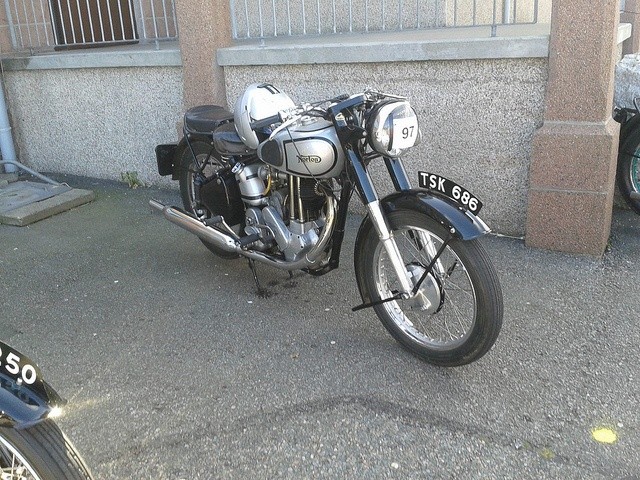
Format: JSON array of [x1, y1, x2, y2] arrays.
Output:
[[613, 97, 640, 216], [0, 340, 94, 480], [150, 83, 503, 367]]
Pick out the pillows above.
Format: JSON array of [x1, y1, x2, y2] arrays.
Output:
[[234, 83, 296, 150]]
